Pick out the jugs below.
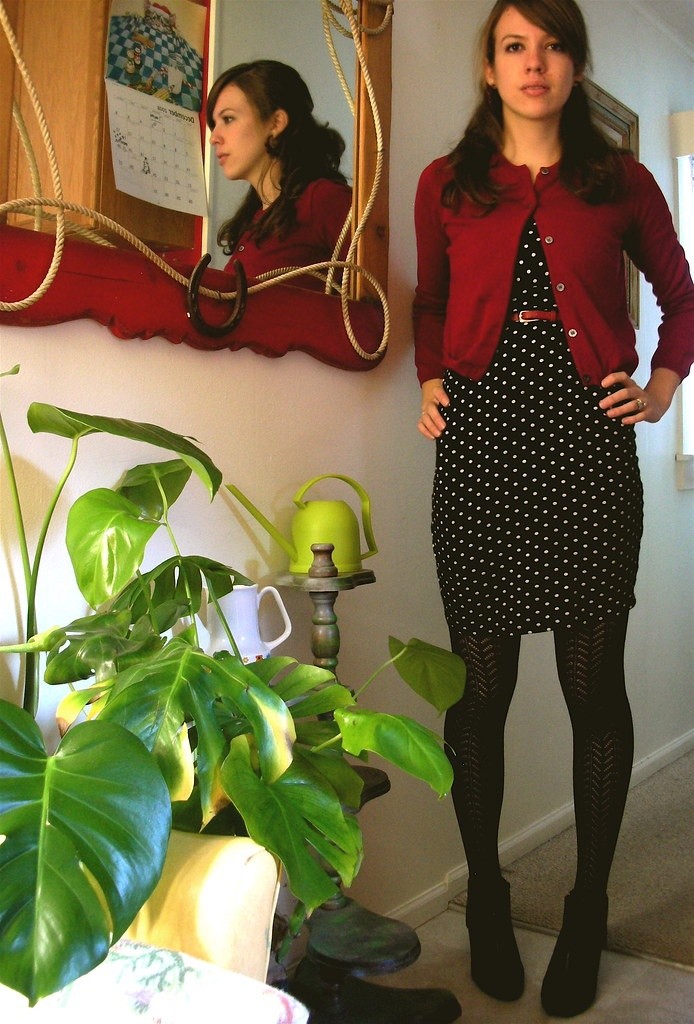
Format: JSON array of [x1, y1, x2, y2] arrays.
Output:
[[179, 583, 294, 667], [224, 470, 379, 575]]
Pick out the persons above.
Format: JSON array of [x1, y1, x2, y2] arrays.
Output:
[[207, 61, 358, 296], [411, 0, 694, 1019]]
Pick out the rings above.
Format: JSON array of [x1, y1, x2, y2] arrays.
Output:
[[421, 412, 425, 415], [636, 398, 647, 410]]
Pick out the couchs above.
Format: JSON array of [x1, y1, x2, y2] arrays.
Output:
[[0, 829, 310, 1024]]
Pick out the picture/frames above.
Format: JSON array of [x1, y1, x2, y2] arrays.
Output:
[[573, 74, 642, 330]]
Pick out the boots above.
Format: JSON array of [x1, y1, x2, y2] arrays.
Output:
[[466, 877, 525, 1002], [540, 887, 610, 1016]]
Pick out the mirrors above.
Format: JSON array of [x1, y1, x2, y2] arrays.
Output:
[[0, 0, 394, 372]]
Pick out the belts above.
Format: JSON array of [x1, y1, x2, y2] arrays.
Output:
[[507, 309, 561, 324]]
